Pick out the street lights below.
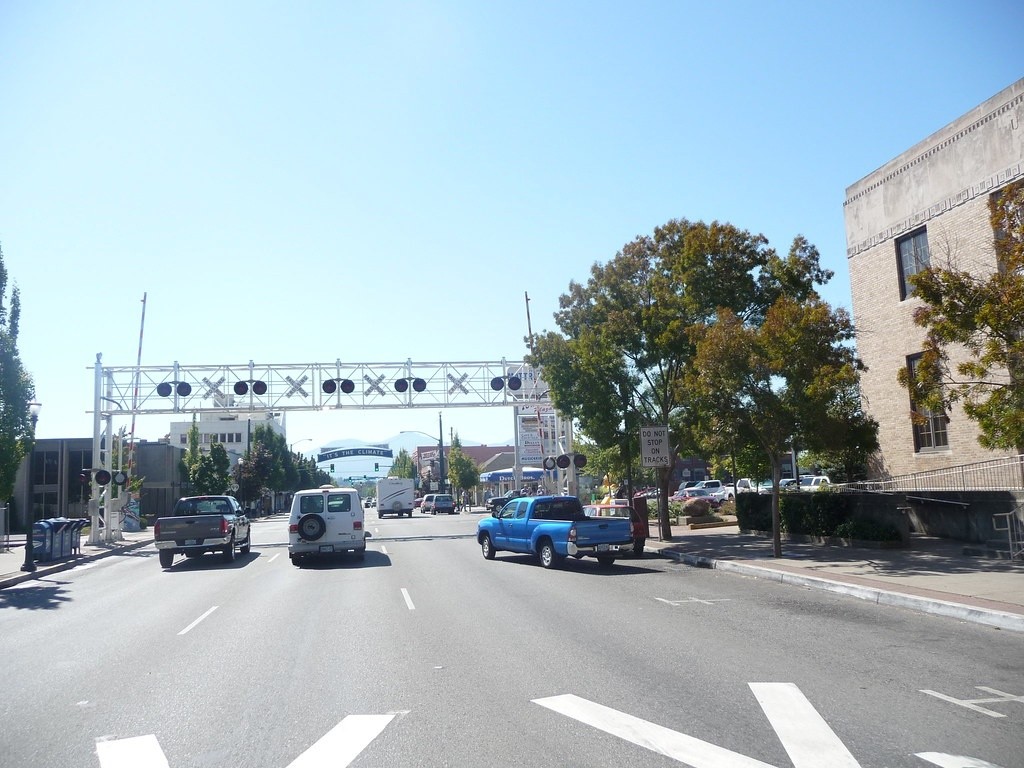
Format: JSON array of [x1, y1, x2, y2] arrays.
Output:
[[238, 457, 244, 502], [290, 439, 312, 478], [399, 431, 446, 495]]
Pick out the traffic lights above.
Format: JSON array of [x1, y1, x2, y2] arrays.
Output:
[[574, 453, 588, 467], [96, 470, 111, 485], [349, 477, 351, 482], [375, 463, 379, 471], [557, 455, 571, 468], [364, 475, 366, 481], [80, 471, 91, 484], [331, 464, 334, 473]]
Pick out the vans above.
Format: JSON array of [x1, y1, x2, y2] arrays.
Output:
[[287, 484, 367, 562]]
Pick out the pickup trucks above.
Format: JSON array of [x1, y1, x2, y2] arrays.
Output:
[[476, 495, 634, 570], [154, 495, 251, 567]]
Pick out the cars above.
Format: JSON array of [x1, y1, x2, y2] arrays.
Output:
[[371, 502, 376, 507], [365, 502, 370, 507], [415, 497, 423, 507], [360, 497, 363, 500], [629, 474, 831, 510], [431, 493, 456, 515], [367, 497, 372, 502], [579, 504, 647, 556], [420, 494, 437, 513]]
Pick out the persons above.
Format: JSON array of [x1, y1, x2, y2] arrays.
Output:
[[615, 483, 624, 499]]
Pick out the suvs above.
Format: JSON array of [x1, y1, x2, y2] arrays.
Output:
[[485, 489, 522, 512]]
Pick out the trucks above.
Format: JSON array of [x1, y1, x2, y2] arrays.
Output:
[[377, 477, 414, 518]]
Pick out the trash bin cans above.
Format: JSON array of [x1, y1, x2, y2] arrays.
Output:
[[632, 497, 649, 537], [32, 517, 91, 565], [600, 497, 610, 516], [610, 499, 630, 518]]
